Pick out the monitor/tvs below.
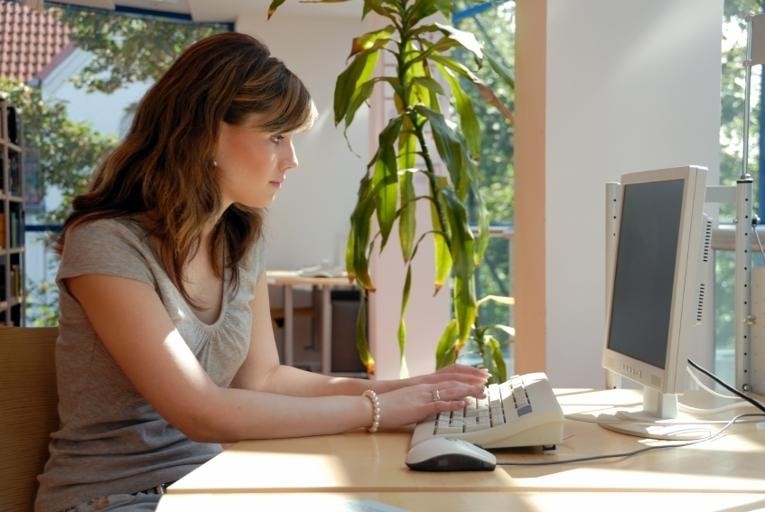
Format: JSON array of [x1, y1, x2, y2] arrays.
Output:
[[596, 166, 716, 440]]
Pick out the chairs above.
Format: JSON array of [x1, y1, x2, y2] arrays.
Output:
[[1, 324, 61, 512]]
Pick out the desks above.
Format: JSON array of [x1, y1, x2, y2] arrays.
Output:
[[161, 385, 764, 490], [272, 272, 365, 379], [154, 490, 764, 512]]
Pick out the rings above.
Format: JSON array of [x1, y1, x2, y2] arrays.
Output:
[[435, 388, 442, 401]]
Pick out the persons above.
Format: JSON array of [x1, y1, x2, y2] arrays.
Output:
[[34, 31, 492, 512]]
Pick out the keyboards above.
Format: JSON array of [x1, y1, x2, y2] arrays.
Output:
[[407, 373, 564, 448]]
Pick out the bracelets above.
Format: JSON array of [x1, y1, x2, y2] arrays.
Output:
[[363, 386, 381, 435]]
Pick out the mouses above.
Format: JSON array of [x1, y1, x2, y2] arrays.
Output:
[[406, 436, 496, 474]]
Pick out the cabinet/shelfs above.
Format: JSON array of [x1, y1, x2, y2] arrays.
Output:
[[1, 95, 31, 329]]
